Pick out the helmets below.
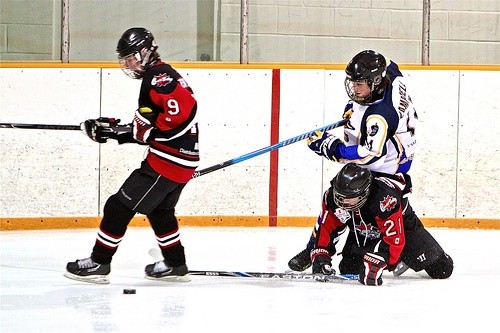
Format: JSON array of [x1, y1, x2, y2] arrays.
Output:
[[116, 27, 158, 80], [344, 50, 386, 104], [331, 163, 372, 211]]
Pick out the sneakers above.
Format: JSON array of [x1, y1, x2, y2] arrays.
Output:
[[290, 249, 314, 274], [64, 256, 112, 284], [144, 260, 191, 282]]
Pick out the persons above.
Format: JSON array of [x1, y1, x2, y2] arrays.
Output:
[[288, 50, 420, 278], [67, 27, 199, 278], [309, 162, 454, 286]]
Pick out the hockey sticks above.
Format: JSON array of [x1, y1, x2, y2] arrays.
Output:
[[0, 122, 134, 132], [189, 117, 349, 180], [148, 247, 383, 286]]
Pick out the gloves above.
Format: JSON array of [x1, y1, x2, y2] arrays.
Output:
[[80, 117, 128, 143], [310, 246, 338, 279], [342, 103, 355, 142], [358, 251, 385, 286], [307, 130, 342, 162]]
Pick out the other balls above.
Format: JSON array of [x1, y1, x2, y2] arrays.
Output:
[[123, 288, 136, 294]]
